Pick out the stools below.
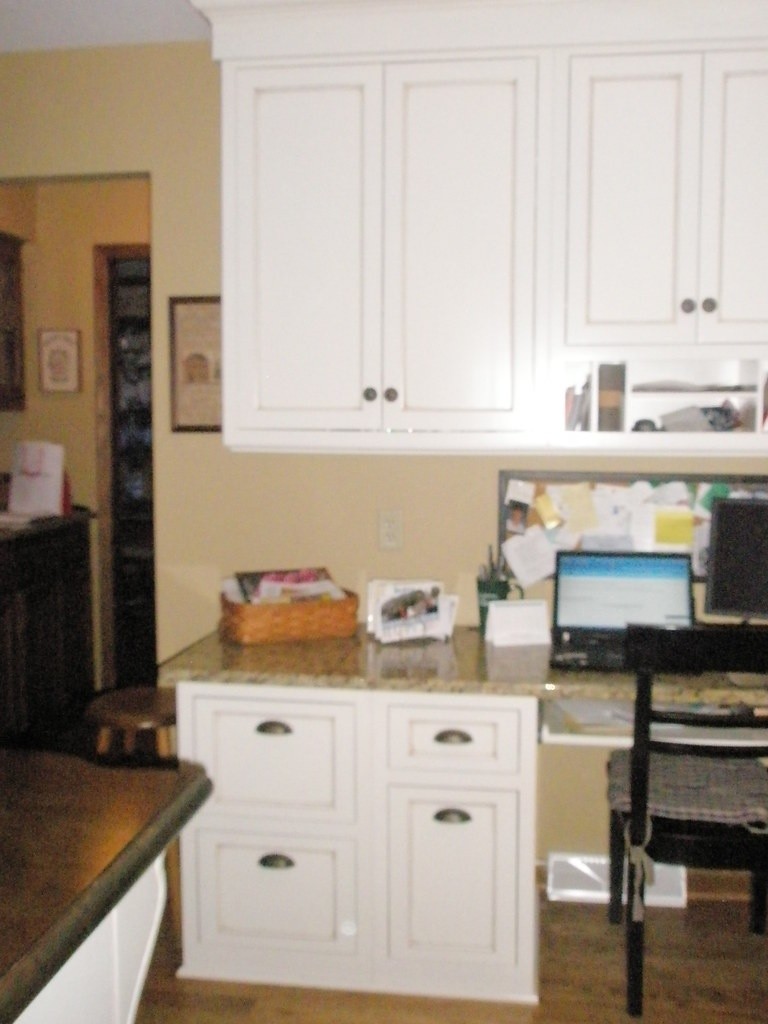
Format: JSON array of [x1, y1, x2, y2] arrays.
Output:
[[87, 688, 180, 945]]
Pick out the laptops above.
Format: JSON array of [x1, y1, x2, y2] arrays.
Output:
[[549, 550, 694, 672]]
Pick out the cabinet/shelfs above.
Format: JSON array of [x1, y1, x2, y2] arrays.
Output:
[[177, 680, 540, 1006], [0, 471, 94, 749], [190, 0, 768, 460]]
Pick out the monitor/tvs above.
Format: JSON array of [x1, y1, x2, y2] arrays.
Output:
[[703, 497, 768, 620]]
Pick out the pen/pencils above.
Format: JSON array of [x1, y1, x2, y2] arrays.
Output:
[[482, 544, 505, 581]]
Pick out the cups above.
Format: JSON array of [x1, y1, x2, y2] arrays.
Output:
[[476, 577, 523, 631]]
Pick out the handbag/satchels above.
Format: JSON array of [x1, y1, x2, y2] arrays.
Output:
[[8, 441, 71, 519]]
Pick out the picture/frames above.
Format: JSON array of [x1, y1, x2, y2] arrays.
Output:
[[169, 296, 221, 432]]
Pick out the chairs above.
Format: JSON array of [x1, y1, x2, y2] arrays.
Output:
[[606, 623, 767, 1018]]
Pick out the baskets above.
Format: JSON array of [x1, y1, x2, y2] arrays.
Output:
[[220, 587, 359, 645]]
